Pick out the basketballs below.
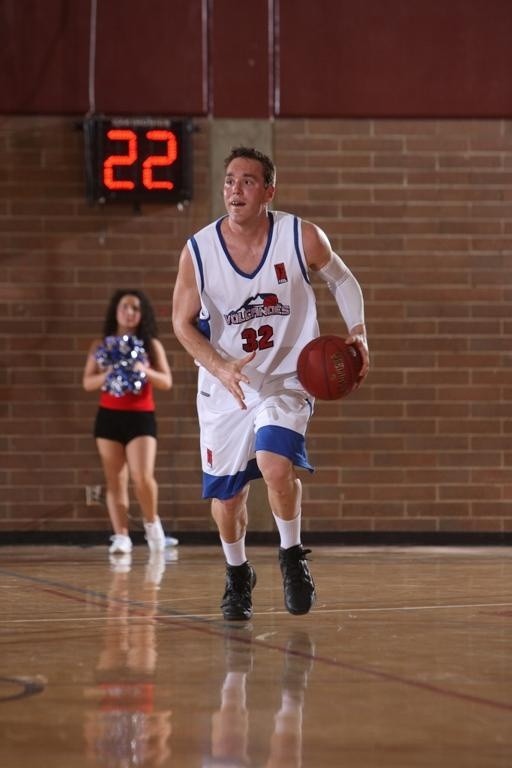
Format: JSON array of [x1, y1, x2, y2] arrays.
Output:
[[296, 335, 362, 400]]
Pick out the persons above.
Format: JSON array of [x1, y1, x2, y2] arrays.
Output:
[[82, 551, 173, 767], [172, 148, 371, 620], [82, 288, 173, 556], [202, 620, 316, 768]]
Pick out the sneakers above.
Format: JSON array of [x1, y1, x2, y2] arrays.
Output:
[[219, 559, 259, 622], [106, 532, 134, 555], [141, 512, 167, 552], [277, 542, 317, 616]]
